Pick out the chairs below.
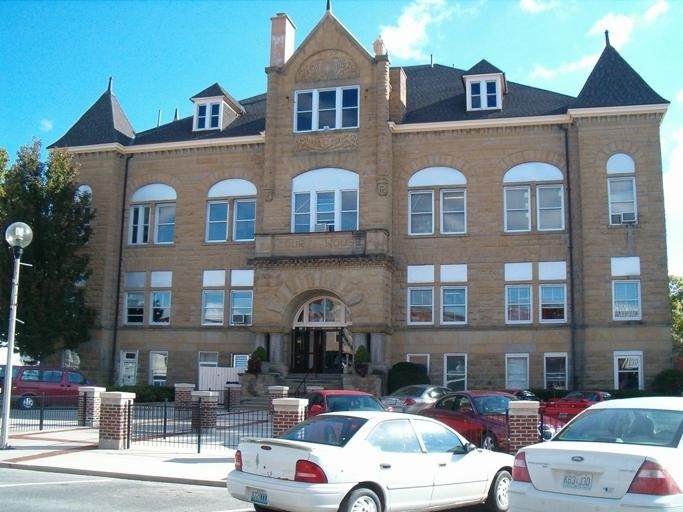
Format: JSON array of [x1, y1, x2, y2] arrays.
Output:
[[629, 416, 655, 440]]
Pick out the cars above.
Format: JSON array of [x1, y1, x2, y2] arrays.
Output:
[[226, 410, 515, 512], [379, 384, 614, 452], [304, 389, 393, 444], [507, 396, 683, 512]]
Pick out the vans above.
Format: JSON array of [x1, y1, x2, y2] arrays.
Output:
[[0, 366, 92, 409]]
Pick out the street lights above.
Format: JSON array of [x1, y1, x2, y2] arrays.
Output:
[[0, 222, 34, 451]]
